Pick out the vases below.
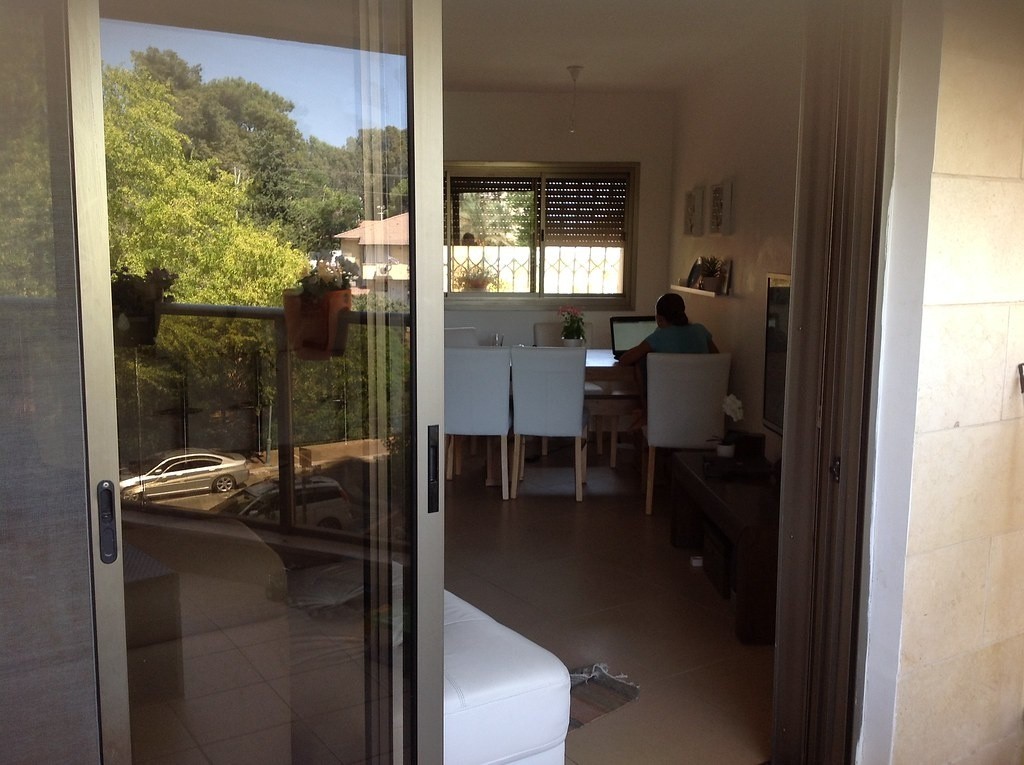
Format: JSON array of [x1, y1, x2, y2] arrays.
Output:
[[717, 445, 735, 457], [282, 289, 351, 362], [562, 339, 583, 347]]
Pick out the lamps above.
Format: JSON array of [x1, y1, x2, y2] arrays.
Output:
[[567, 65, 582, 133]]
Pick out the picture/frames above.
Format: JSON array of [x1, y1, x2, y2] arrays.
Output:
[[682, 187, 704, 237], [707, 182, 731, 237]]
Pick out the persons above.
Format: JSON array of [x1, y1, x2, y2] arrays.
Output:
[[619, 295, 720, 435]]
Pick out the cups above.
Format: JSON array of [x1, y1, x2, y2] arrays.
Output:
[[490, 333, 504, 346]]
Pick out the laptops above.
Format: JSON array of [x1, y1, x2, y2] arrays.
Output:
[[610, 315, 658, 360]]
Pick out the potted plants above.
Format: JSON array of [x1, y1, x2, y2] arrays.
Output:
[[456, 269, 502, 292], [112, 265, 179, 344], [698, 255, 721, 294]]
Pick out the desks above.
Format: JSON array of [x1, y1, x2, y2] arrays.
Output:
[[670, 451, 781, 645], [584, 348, 644, 383]]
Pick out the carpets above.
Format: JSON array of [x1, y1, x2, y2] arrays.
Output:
[[568, 663, 641, 733]]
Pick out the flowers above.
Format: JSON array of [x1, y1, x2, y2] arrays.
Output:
[[559, 304, 588, 342], [296, 248, 360, 296], [706, 395, 744, 445]]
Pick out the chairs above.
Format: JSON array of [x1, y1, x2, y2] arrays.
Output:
[[444, 322, 732, 515]]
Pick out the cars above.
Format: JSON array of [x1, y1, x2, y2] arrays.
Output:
[[210, 475, 352, 531], [119, 447, 249, 504]]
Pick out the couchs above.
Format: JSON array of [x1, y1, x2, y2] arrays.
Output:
[[117, 516, 572, 763]]
